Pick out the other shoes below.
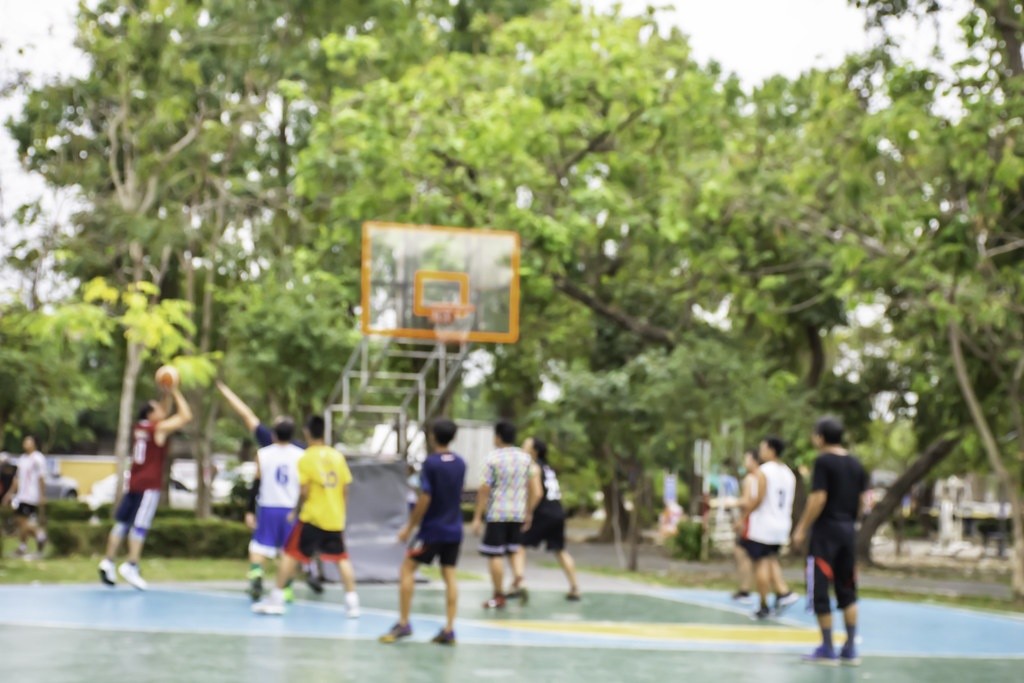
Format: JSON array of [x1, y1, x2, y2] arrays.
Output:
[[98, 559, 116, 584], [565, 593, 581, 602], [753, 603, 770, 620], [801, 650, 837, 666], [483, 594, 506, 608], [836, 653, 861, 665], [432, 630, 456, 646], [251, 602, 283, 615], [772, 592, 799, 612], [118, 562, 147, 590], [379, 624, 412, 643], [344, 606, 361, 621]]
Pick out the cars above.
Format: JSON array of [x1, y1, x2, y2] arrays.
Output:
[[9, 458, 80, 499], [93, 460, 232, 509]]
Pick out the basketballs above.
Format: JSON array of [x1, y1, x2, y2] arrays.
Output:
[[154, 364, 180, 389]]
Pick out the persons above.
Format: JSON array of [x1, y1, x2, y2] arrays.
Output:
[[378, 417, 468, 645], [244, 421, 305, 603], [1, 434, 49, 557], [792, 414, 871, 666], [729, 437, 798, 618], [217, 381, 324, 593], [472, 421, 579, 610], [249, 415, 361, 619], [97, 384, 193, 591]]
